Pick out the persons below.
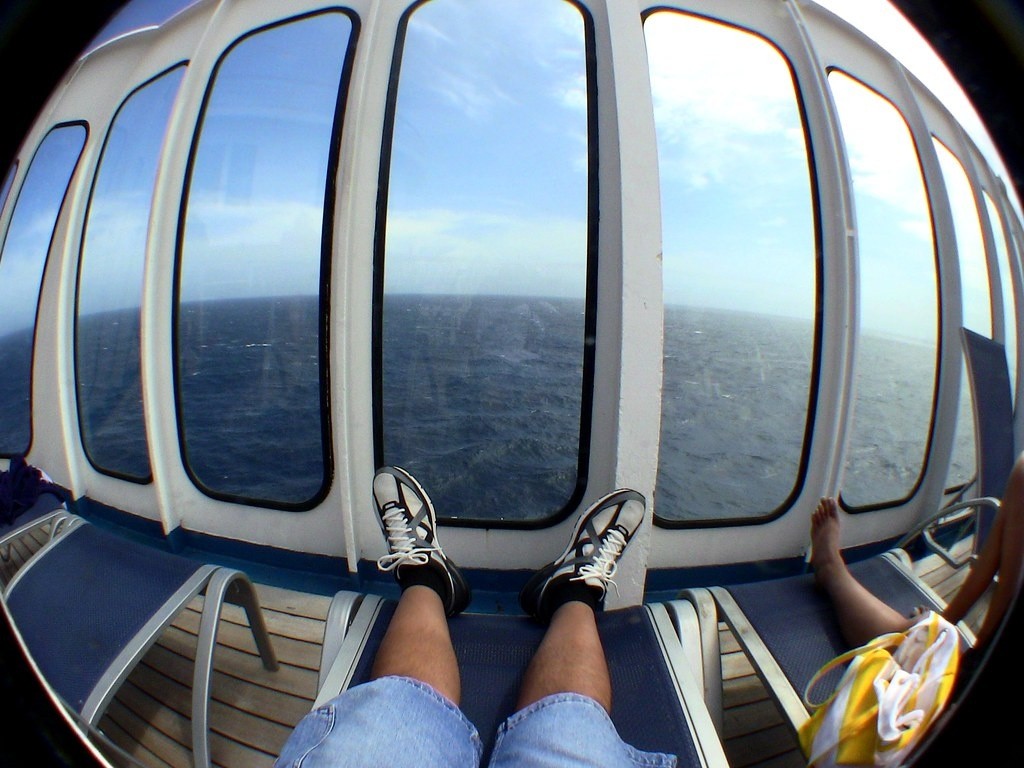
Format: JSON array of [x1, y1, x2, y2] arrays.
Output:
[[811, 452, 1023, 727], [274, 465, 677, 766]]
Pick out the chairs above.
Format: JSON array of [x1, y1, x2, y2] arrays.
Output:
[[0, 514, 279, 768], [684, 552, 1024, 768], [0, 490, 69, 561], [310, 591, 727, 767]]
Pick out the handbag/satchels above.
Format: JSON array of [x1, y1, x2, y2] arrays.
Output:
[[796, 609, 960, 768]]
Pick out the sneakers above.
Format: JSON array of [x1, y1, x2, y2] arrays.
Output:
[[371, 466, 471, 619], [519, 488, 646, 620]]
